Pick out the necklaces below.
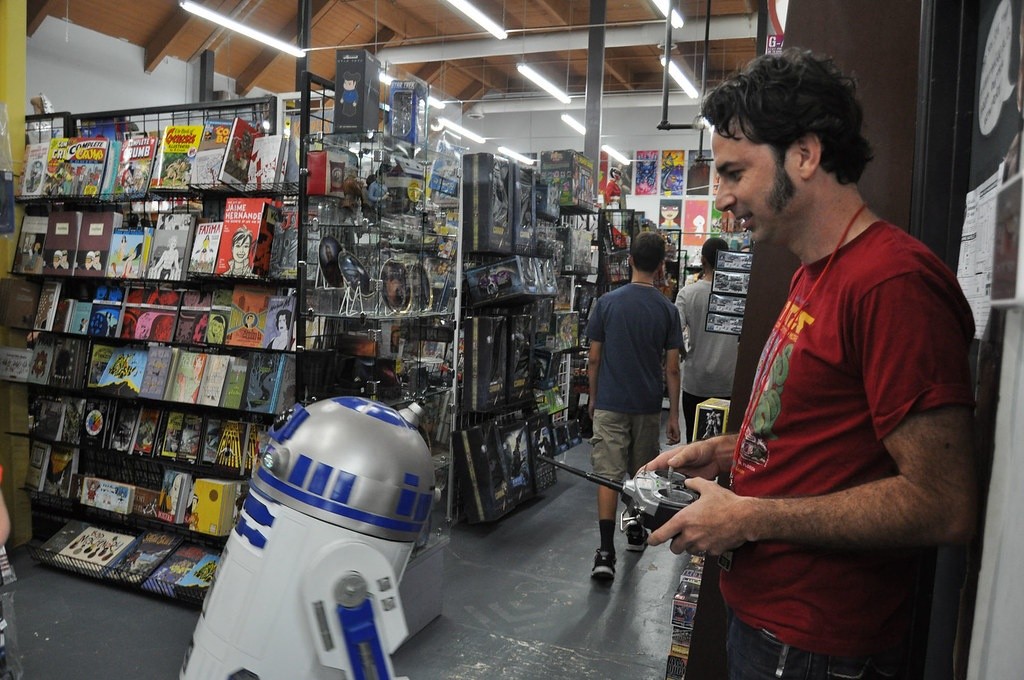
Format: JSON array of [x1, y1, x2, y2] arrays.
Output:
[[631, 280, 656, 287]]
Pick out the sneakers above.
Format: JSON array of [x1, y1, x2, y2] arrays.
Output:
[[591, 546, 617, 579], [625, 525, 649, 552]]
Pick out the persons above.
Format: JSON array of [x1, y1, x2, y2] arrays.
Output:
[[0, 466, 25, 680], [584, 231, 689, 580], [674, 237, 739, 445], [633, 45, 983, 680]]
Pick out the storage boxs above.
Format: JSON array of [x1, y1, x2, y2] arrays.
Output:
[[463, 149, 594, 524], [389, 80, 430, 161], [334, 48, 381, 143], [692, 398, 731, 443]]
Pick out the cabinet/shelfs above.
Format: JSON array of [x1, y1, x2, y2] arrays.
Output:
[[598, 209, 687, 305], [6, 95, 338, 610], [295, 70, 463, 649]]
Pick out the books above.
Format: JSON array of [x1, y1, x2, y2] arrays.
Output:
[[21, 117, 290, 198], [13, 210, 223, 282], [24, 284, 299, 599]]
